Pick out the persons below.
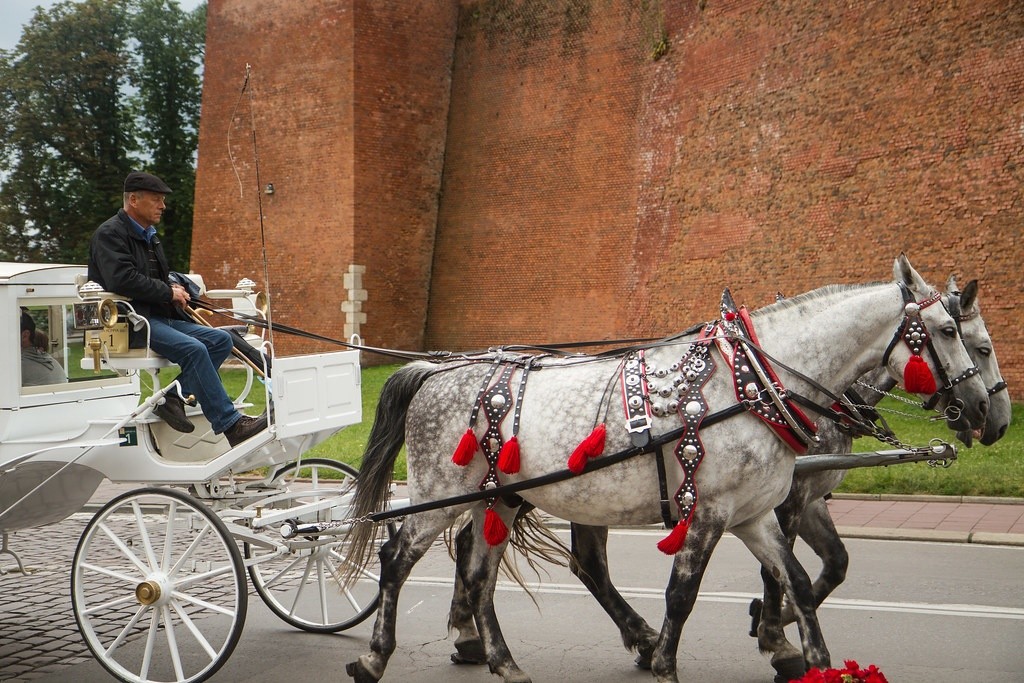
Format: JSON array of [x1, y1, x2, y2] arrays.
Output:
[[87, 172, 274, 448], [20, 312, 69, 387]]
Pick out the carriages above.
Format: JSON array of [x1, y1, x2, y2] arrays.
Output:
[[0, 254, 1013, 683]]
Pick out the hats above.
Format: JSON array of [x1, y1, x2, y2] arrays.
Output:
[[125, 173, 173, 193]]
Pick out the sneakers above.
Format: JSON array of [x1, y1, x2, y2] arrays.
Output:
[[152, 394, 195, 433], [224, 415, 273, 449]]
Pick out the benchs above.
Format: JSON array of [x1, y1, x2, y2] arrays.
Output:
[[77, 273, 269, 416]]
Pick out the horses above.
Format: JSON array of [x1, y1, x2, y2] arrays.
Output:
[[332, 252, 991, 682], [447, 273, 1011, 683]]
